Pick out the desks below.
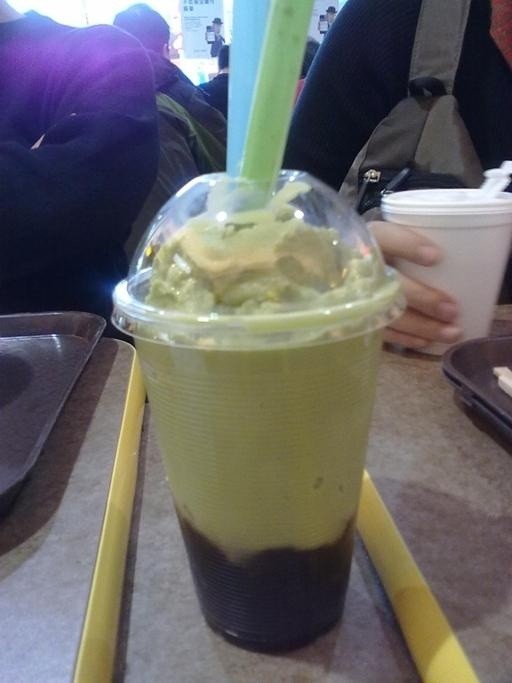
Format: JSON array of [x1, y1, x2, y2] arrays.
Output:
[[0, 301, 511, 682]]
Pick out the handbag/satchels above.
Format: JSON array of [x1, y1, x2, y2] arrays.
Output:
[[331, 0, 487, 224]]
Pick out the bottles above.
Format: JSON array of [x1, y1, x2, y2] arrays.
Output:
[[206, 26, 217, 44], [319, 15, 329, 34]]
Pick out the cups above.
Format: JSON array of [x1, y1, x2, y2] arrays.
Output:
[[379, 186, 512, 355]]
